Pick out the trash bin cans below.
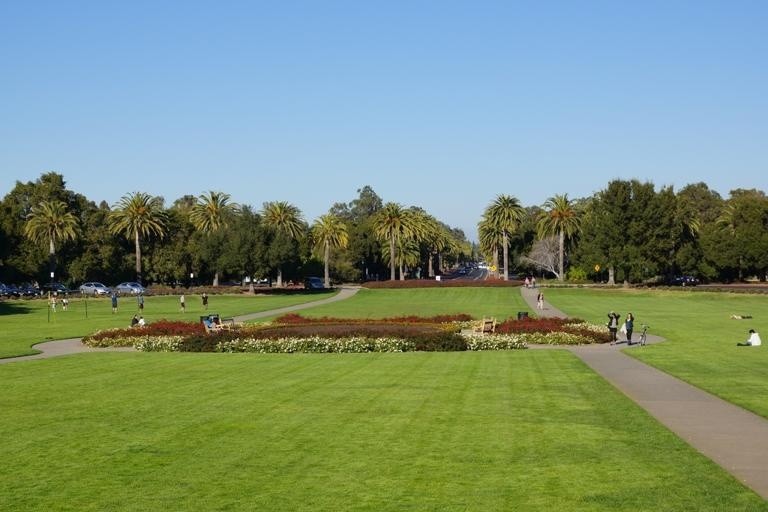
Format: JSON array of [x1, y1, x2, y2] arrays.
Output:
[[518, 312, 528, 319], [222, 318, 234, 325]]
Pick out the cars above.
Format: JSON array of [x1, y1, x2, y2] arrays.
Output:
[[304, 276, 323, 290], [114, 282, 146, 294], [666, 275, 697, 286], [0, 282, 72, 297], [79, 282, 111, 295], [222, 280, 241, 286], [246, 276, 268, 283]]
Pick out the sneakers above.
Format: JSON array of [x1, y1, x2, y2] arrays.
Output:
[[610, 340, 632, 346]]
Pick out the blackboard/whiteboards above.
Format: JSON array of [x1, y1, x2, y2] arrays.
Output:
[[483, 319, 495, 331]]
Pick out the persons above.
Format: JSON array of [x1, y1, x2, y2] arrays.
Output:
[[112, 292, 118, 313], [525, 276, 536, 290], [737, 330, 763, 348], [132, 315, 145, 326], [730, 314, 752, 319], [202, 293, 208, 310], [180, 295, 185, 314], [48, 289, 68, 311], [536, 293, 544, 310], [138, 293, 144, 310], [606, 311, 635, 346]]
